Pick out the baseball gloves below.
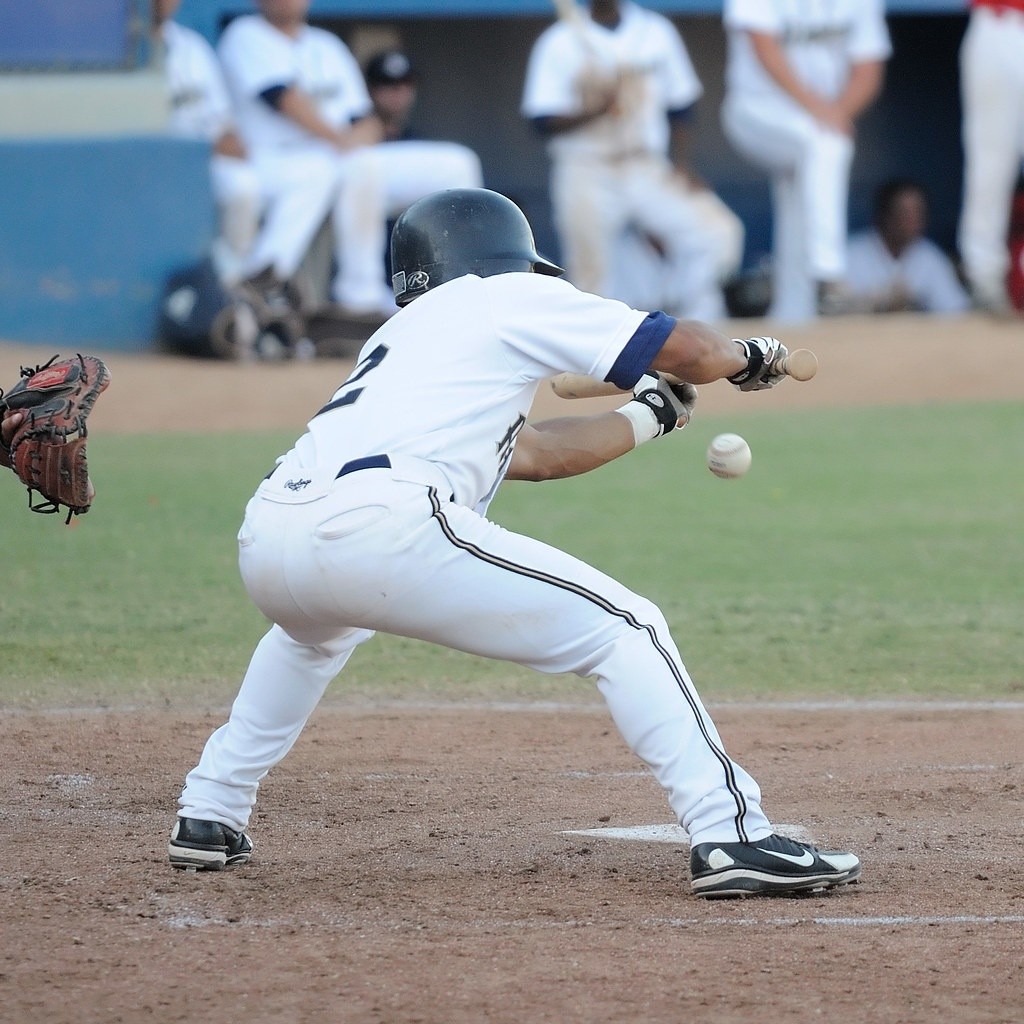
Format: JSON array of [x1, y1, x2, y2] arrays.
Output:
[[0, 356, 111, 515]]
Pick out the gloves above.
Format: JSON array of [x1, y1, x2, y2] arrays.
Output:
[[726, 336, 790, 393], [631, 363, 699, 439]]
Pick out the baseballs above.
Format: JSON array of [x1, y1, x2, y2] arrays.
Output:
[[703, 431, 753, 480]]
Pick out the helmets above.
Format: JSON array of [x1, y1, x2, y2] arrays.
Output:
[[389, 188, 564, 307]]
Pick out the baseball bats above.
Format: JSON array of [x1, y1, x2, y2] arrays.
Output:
[[551, 0, 630, 166], [550, 347, 820, 401]]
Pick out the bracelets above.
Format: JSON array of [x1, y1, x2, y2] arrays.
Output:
[[614, 400, 670, 449]]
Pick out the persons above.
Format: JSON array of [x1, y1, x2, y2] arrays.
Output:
[[1, 351, 113, 526], [148, 0, 1023, 365], [169, 190, 860, 901]]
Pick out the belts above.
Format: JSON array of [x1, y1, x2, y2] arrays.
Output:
[[263, 454, 390, 478]]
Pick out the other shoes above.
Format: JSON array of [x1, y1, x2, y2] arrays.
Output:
[[219, 257, 409, 364]]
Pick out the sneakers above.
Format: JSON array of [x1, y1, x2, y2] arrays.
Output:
[[688, 830, 862, 898], [166, 817, 253, 870]]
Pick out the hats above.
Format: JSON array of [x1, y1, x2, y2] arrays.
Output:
[[364, 47, 420, 88]]
